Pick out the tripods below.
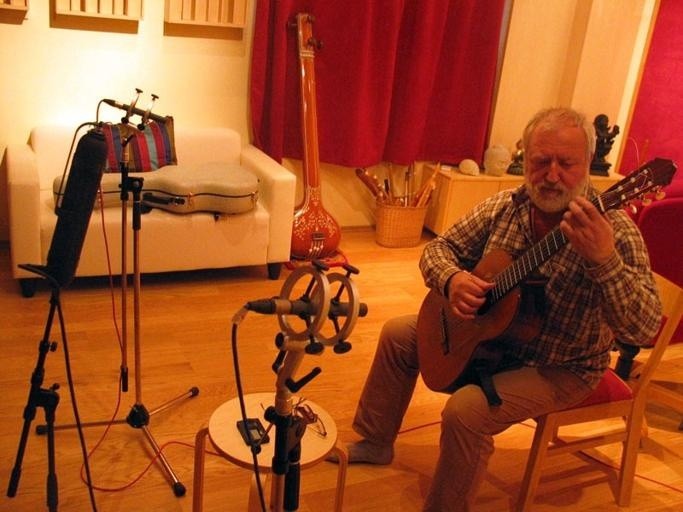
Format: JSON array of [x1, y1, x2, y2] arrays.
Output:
[[35, 209, 200, 498]]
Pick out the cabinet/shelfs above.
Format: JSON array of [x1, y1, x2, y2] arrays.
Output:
[[422, 164, 627, 238]]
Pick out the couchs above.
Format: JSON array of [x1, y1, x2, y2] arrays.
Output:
[[4, 119, 299, 297]]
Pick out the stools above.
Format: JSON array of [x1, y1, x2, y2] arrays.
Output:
[[191, 390, 348, 512]]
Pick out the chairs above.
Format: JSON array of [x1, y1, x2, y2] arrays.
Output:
[[611, 198, 683, 380], [515, 265, 683, 512]]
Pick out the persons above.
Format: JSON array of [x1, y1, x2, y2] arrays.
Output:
[[322, 102, 664, 512]]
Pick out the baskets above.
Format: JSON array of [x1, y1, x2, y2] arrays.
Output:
[[376, 202, 428, 247]]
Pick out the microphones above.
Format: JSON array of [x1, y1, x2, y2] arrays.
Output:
[[104, 86, 168, 133], [248, 258, 370, 357], [43, 119, 110, 303]]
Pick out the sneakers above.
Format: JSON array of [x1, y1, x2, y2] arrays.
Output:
[[326, 439, 394, 464]]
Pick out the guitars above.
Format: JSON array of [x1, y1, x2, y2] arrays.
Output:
[[414, 157, 680, 395]]
